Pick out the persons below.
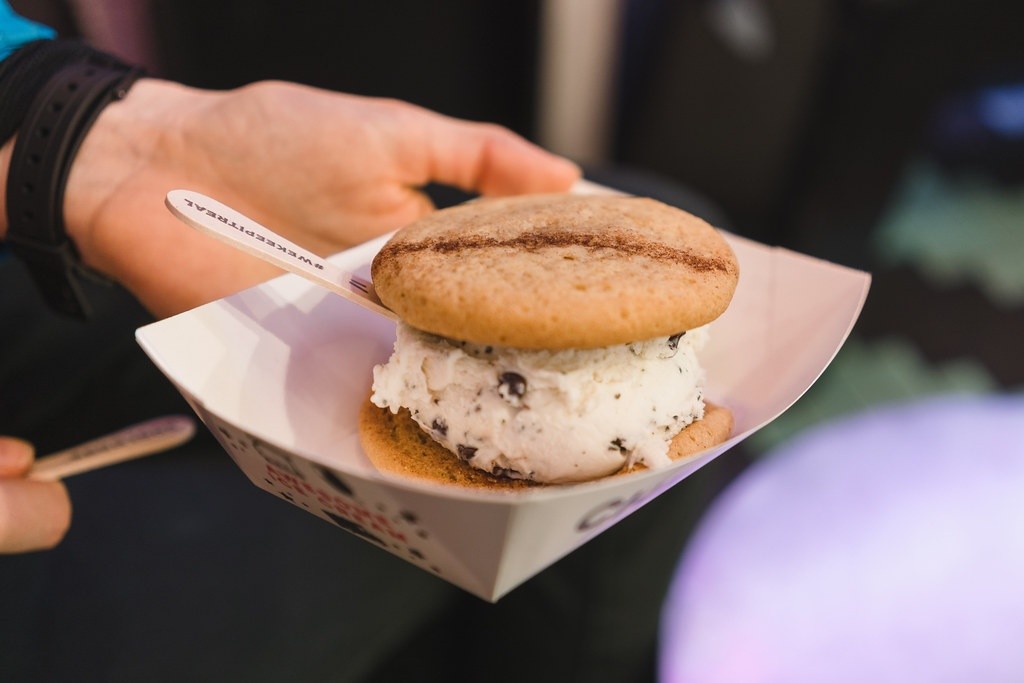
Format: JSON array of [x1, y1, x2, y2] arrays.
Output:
[[0, 0, 582, 557]]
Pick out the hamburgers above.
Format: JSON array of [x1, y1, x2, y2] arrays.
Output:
[[361, 191, 740, 495]]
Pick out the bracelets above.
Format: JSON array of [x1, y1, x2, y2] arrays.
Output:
[[4, 56, 146, 322]]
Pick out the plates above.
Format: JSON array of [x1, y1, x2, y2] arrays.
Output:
[[656, 396, 1023, 683]]
